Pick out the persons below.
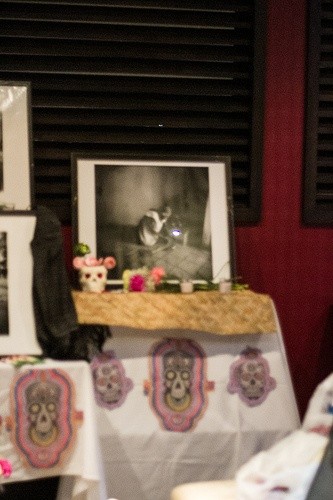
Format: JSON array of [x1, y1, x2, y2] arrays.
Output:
[[134, 205, 178, 248]]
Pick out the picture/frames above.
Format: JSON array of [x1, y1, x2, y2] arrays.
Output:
[[0, 208, 50, 363], [0, 80, 36, 217], [65, 149, 237, 295]]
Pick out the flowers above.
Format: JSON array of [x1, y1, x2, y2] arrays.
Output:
[[116, 265, 163, 294]]
